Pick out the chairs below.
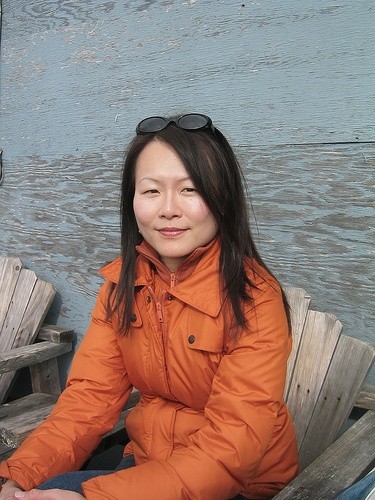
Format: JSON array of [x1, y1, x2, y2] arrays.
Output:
[[1, 255, 76, 486], [100, 283, 375, 500]]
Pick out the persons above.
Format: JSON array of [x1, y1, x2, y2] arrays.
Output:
[[1, 113, 301, 500]]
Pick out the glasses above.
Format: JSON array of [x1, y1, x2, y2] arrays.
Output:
[[135, 113, 217, 135]]
